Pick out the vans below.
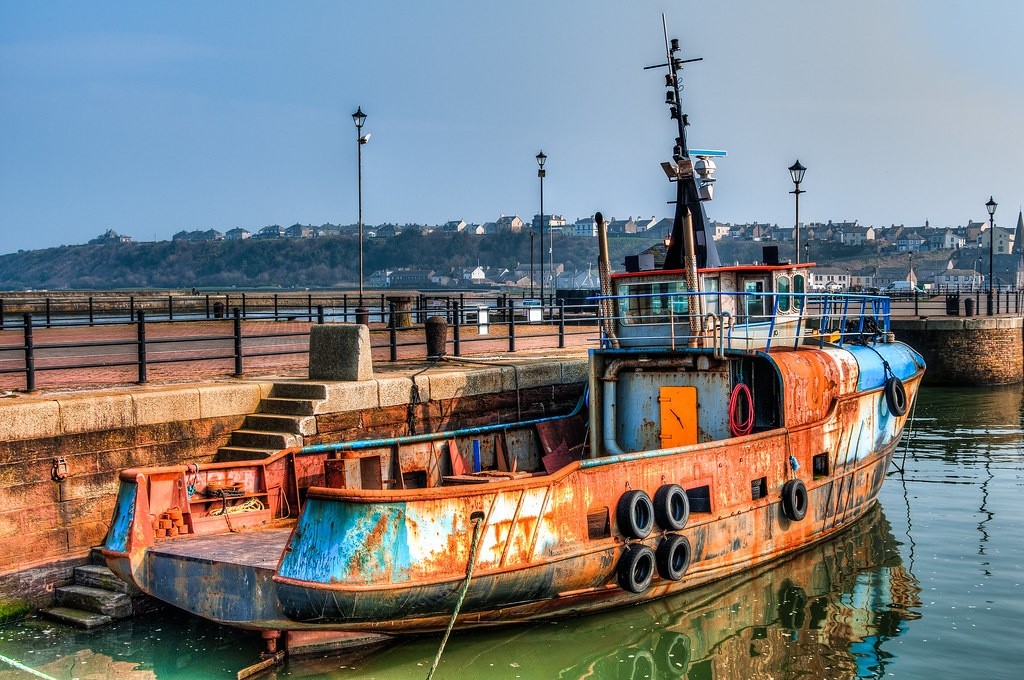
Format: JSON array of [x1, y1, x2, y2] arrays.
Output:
[[882, 280, 915, 297]]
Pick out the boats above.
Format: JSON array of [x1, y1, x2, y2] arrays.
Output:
[[101, 291, 928, 626]]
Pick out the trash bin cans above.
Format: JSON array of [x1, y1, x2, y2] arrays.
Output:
[[945, 292, 959, 315]]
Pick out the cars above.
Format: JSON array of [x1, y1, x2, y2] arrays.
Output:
[[914, 286, 928, 296]]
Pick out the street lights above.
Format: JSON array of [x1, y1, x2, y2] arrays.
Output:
[[537, 149, 549, 306], [352, 107, 367, 303], [979, 256, 982, 286], [846, 269, 849, 289], [986, 196, 997, 295], [1006, 268, 1008, 285], [908, 251, 913, 291], [790, 160, 807, 264]]
